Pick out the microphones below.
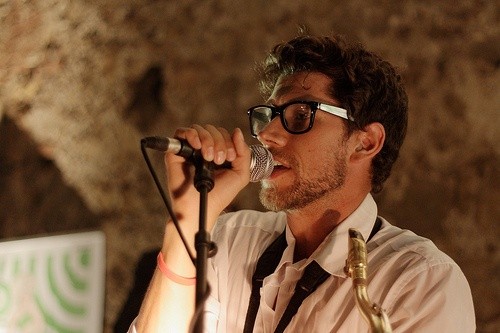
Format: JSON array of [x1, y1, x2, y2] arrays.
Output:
[[143, 136, 274, 182]]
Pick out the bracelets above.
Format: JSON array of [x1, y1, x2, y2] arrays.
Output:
[[157, 250, 196, 286]]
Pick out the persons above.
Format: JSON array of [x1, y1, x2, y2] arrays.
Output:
[[125, 33, 476, 333]]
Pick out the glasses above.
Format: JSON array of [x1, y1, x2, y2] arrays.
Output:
[[247, 100, 355, 136]]
